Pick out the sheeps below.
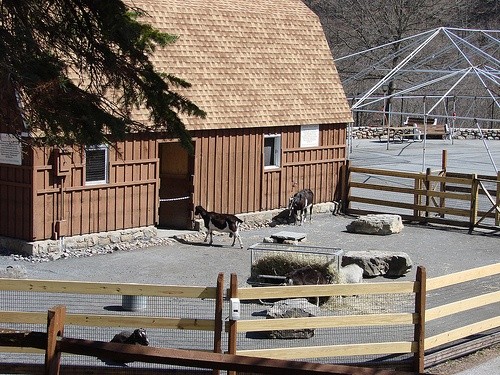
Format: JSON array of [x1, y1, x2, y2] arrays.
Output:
[[290, 189, 314, 226], [195, 206, 244, 249], [110, 327, 150, 346]]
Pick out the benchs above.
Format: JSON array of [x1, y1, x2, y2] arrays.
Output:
[[413, 123, 449, 140]]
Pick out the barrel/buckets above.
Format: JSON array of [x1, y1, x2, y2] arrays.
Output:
[[122, 295, 146, 311]]
